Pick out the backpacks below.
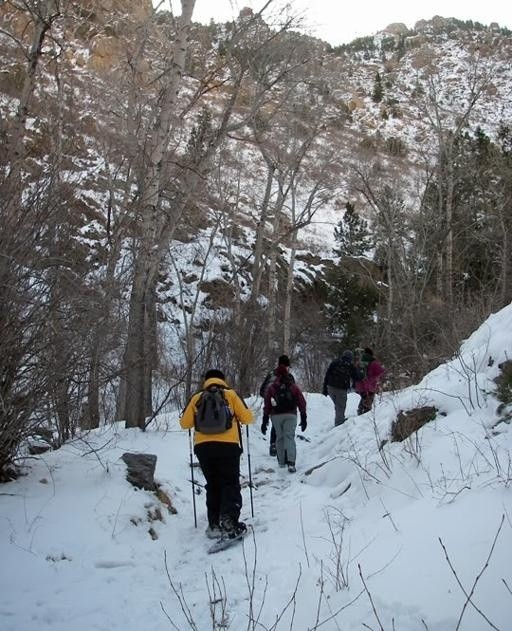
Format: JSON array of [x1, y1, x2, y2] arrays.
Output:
[[358, 361, 369, 381], [195, 385, 231, 435], [265, 377, 277, 396], [272, 385, 294, 414]]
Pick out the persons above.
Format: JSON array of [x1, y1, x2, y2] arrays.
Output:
[[180, 369, 255, 542], [322, 349, 360, 427], [261, 365, 307, 472], [354, 348, 391, 416], [260, 354, 295, 458]]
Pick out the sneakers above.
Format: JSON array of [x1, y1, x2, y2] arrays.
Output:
[[219, 498, 245, 538], [205, 505, 222, 540], [269, 444, 295, 472]]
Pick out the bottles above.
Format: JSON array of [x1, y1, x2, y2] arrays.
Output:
[[270, 396, 277, 407], [224, 403, 230, 426]]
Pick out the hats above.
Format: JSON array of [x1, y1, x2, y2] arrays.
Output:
[[365, 348, 373, 354], [278, 355, 291, 367], [205, 369, 226, 380], [343, 350, 353, 356]]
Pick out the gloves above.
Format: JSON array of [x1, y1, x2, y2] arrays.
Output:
[[261, 418, 268, 434], [298, 416, 307, 432], [322, 388, 329, 396]]
